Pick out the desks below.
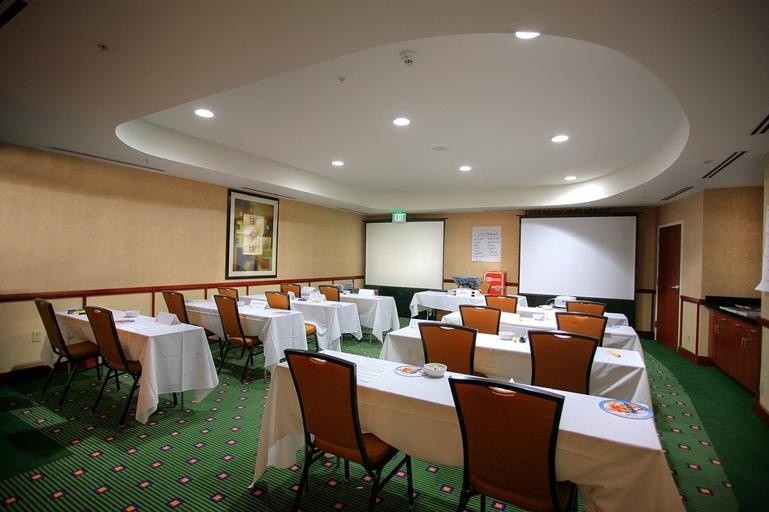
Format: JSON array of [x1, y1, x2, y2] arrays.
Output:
[[516, 305, 628, 326], [247, 350, 686, 512], [444, 310, 643, 352], [380, 326, 652, 409]]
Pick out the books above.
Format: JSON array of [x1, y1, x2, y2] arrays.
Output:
[[719, 304, 760, 317]]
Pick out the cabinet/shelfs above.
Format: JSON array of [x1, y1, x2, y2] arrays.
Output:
[[708, 309, 757, 393]]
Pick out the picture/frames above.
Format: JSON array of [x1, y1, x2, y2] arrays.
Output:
[[225, 187, 280, 278]]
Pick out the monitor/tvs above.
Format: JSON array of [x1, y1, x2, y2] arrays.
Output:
[[453, 275, 482, 288]]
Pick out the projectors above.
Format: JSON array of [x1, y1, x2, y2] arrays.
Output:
[[357, 289, 380, 296], [554, 296, 577, 307]]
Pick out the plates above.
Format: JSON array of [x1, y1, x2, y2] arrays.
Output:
[[599, 398, 654, 420], [395, 365, 426, 377]]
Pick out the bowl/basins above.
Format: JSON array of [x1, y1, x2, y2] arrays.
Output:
[[124, 310, 141, 317], [532, 305, 553, 321], [236, 301, 246, 306], [498, 331, 515, 340], [424, 363, 448, 377]]
